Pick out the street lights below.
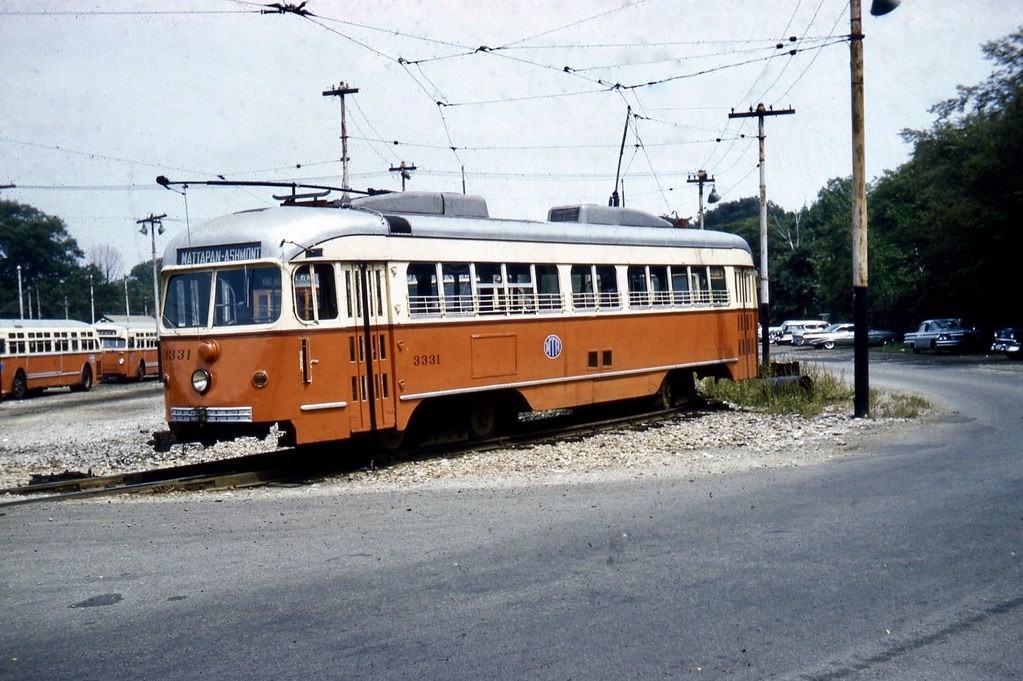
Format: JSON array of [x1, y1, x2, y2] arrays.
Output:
[[135, 214, 167, 382], [849, 0, 902, 416]]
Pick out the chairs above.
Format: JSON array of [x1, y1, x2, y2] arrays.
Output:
[[673, 275, 696, 303], [702, 279, 724, 302]]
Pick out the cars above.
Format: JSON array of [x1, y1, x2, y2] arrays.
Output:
[[990, 326, 1023, 358], [904, 319, 972, 355], [776, 320, 831, 345], [791, 323, 898, 349]]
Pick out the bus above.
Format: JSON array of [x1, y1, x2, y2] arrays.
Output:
[[90, 323, 159, 384], [144, 192, 760, 456], [0, 319, 104, 402]]
